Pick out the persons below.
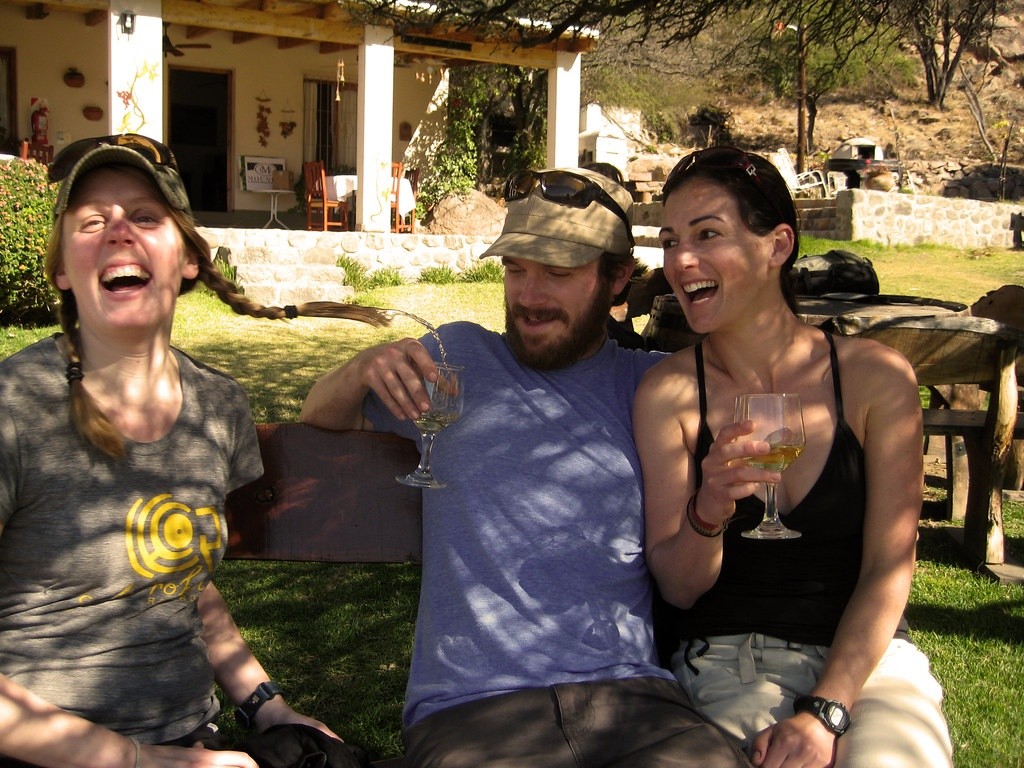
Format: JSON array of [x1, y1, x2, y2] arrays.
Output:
[[630, 145, 954, 768], [0, 133, 369, 768], [295, 161, 754, 768]]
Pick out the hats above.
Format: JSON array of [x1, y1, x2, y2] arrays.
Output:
[[478, 168, 634, 268], [53, 144, 191, 222]]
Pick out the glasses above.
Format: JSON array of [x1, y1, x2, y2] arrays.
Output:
[[45, 133, 179, 212], [659, 146, 781, 215], [502, 169, 636, 248]]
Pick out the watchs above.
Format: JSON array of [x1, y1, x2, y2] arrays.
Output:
[[234, 682, 286, 730], [793, 695, 850, 733]]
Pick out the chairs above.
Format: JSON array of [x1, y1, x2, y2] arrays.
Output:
[[767, 148, 831, 199], [847, 284, 1024, 582], [303, 162, 422, 234]]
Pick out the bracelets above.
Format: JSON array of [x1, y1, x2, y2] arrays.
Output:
[[129, 736, 141, 768], [687, 489, 736, 537]]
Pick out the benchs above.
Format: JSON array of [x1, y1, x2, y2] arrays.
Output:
[[224, 424, 421, 564]]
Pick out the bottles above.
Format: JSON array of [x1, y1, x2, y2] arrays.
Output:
[[273, 164, 289, 190]]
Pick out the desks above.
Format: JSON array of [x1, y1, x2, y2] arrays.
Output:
[[251, 189, 296, 230], [315, 176, 414, 229], [641, 295, 1004, 519]]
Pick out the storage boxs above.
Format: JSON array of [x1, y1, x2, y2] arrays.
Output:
[[273, 170, 294, 190]]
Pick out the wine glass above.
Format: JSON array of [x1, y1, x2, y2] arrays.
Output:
[[394, 362, 464, 488], [732, 394, 806, 539]]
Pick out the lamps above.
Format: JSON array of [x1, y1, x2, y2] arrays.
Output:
[[162, 22, 184, 59], [121, 11, 136, 34], [336, 42, 345, 101], [412, 56, 445, 84]]
